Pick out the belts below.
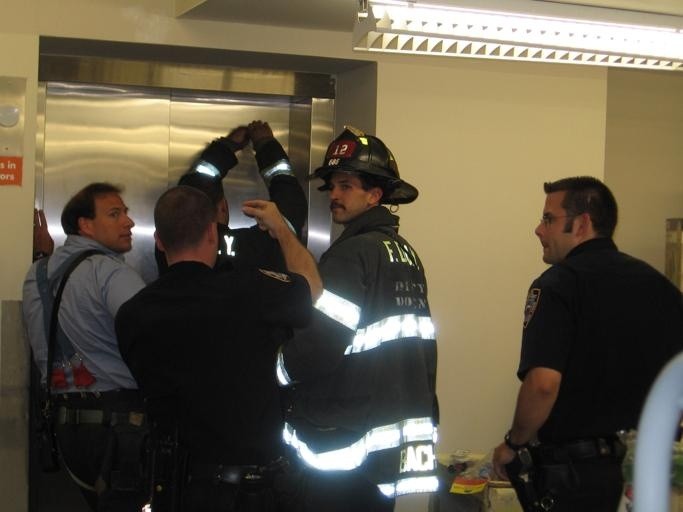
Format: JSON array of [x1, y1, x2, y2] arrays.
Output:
[[54, 390, 145, 409], [214, 458, 290, 487]]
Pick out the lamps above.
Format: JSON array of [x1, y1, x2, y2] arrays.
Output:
[[345, 0, 683, 80]]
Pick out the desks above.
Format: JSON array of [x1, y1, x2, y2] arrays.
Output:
[[426, 451, 682, 512]]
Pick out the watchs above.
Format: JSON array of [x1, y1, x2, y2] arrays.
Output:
[[502, 429, 530, 451], [34, 251, 46, 260]]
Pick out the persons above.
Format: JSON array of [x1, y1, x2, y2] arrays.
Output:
[[275, 129, 440, 512], [153, 121, 308, 284], [492, 176, 683, 512], [114, 185, 323, 512], [22, 182, 147, 512]]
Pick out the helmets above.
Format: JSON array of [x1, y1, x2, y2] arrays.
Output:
[[315, 127, 418, 205]]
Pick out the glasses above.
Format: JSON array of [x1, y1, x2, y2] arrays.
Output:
[[543, 212, 584, 227]]
